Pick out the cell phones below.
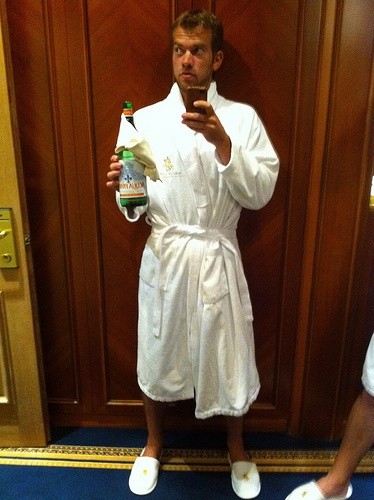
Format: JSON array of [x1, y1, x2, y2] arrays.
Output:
[[186, 86, 208, 115]]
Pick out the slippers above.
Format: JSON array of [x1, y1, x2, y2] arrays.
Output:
[[129, 445, 159, 495], [228, 453, 262, 498], [286, 480, 353, 500]]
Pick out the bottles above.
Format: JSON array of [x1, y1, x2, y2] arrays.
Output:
[[116, 101, 149, 207]]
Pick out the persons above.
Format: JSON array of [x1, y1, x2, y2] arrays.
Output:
[[285, 175, 374, 500], [104, 9, 281, 499]]
